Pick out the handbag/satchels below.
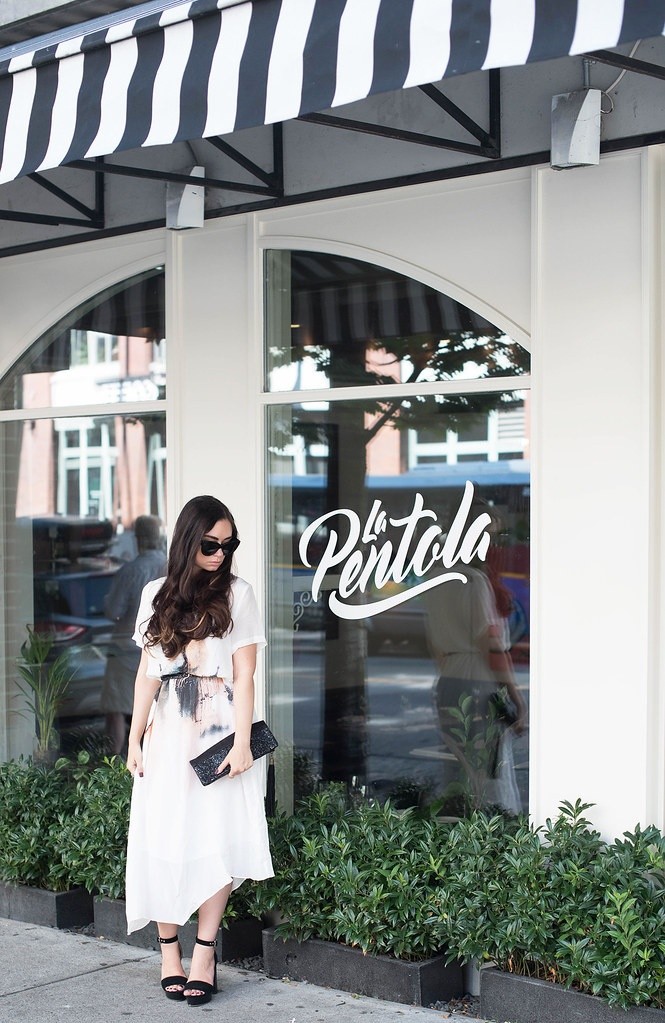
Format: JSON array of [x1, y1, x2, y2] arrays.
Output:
[[189, 720, 279, 819], [489, 691, 520, 779]]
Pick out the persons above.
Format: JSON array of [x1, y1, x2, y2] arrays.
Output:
[[99, 514, 167, 760], [417, 492, 530, 817], [124, 494, 276, 1005]]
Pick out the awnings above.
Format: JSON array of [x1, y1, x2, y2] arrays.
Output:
[[0, 0, 664, 255]]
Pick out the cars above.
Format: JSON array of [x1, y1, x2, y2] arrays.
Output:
[[28, 514, 135, 758], [363, 578, 433, 660]]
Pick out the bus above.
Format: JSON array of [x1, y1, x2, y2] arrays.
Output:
[[268, 462, 530, 665]]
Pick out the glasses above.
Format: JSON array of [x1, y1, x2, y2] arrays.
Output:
[[200, 537, 241, 556]]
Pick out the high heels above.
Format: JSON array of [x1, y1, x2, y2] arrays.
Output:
[[183, 938, 218, 1007], [156, 934, 188, 1001]]
